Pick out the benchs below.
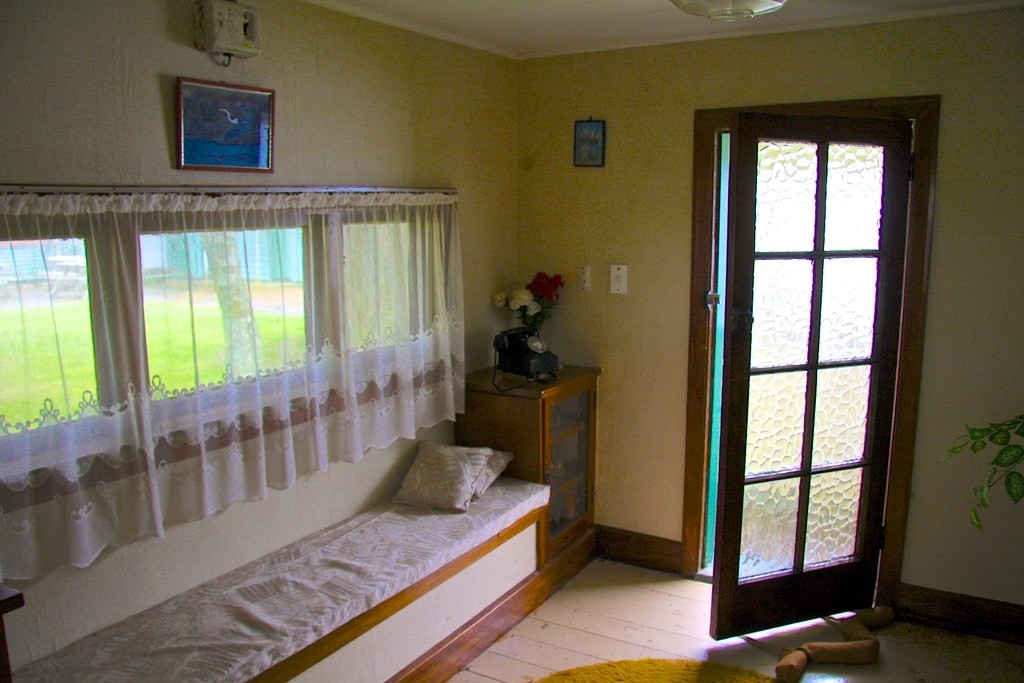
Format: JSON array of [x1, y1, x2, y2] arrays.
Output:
[[12, 473, 553, 683]]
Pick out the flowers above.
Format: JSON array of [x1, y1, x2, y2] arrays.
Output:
[[492, 272, 564, 328]]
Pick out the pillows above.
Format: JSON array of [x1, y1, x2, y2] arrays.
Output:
[[391, 440, 493, 512], [470, 450, 514, 503]]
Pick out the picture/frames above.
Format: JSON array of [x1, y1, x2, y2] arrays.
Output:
[[574, 120, 605, 167], [177, 76, 276, 173]]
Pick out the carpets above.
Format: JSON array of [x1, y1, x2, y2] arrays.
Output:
[[533, 657, 775, 683]]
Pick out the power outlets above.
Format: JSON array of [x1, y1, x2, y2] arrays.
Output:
[[574, 265, 592, 293]]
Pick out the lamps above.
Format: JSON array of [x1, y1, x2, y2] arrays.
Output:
[[672, 0, 787, 21]]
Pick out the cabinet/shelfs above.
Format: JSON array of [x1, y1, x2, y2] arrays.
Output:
[[454, 364, 601, 561]]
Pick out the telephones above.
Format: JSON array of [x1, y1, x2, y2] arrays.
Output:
[[493, 327, 558, 380]]
